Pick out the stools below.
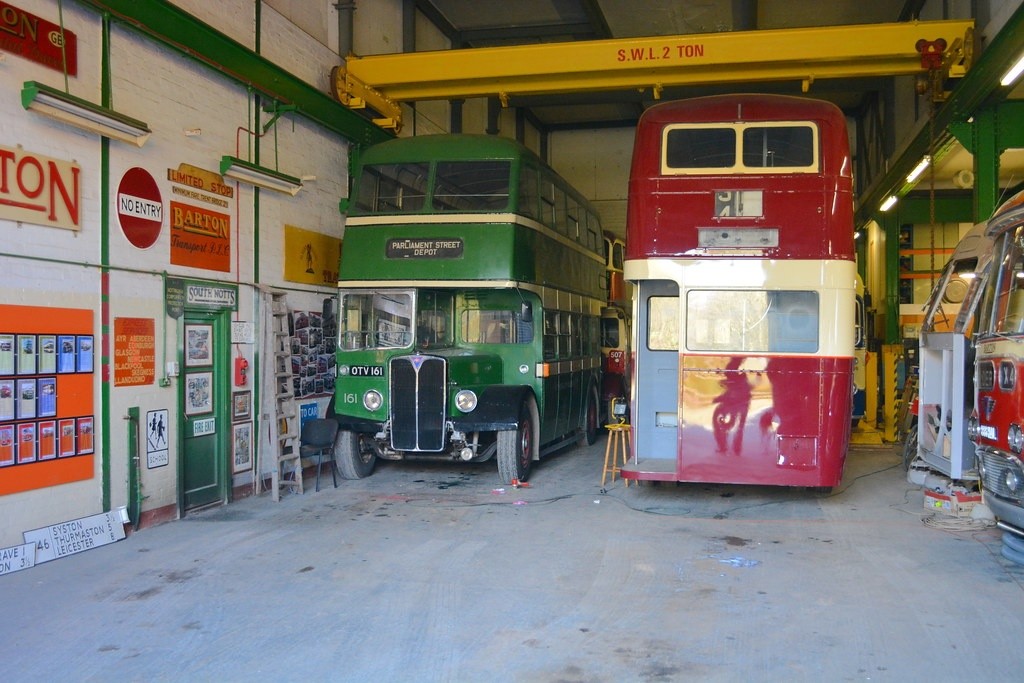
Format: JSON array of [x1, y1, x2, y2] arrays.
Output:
[[601, 425, 639, 487]]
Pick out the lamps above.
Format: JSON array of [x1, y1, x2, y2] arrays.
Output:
[[21, 0, 152, 148], [904, 155, 931, 183], [219, 87, 302, 199]]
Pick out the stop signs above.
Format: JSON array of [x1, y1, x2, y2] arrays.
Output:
[[115, 166, 164, 250]]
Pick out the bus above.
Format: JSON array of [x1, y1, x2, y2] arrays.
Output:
[[618, 92, 860, 497], [331, 132, 610, 486], [601, 228, 632, 425], [851, 273, 876, 429], [966, 184, 1024, 539]]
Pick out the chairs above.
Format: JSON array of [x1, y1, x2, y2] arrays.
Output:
[[281, 419, 340, 492]]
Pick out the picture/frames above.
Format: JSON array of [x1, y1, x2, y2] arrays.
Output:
[[0, 333, 94, 468]]
